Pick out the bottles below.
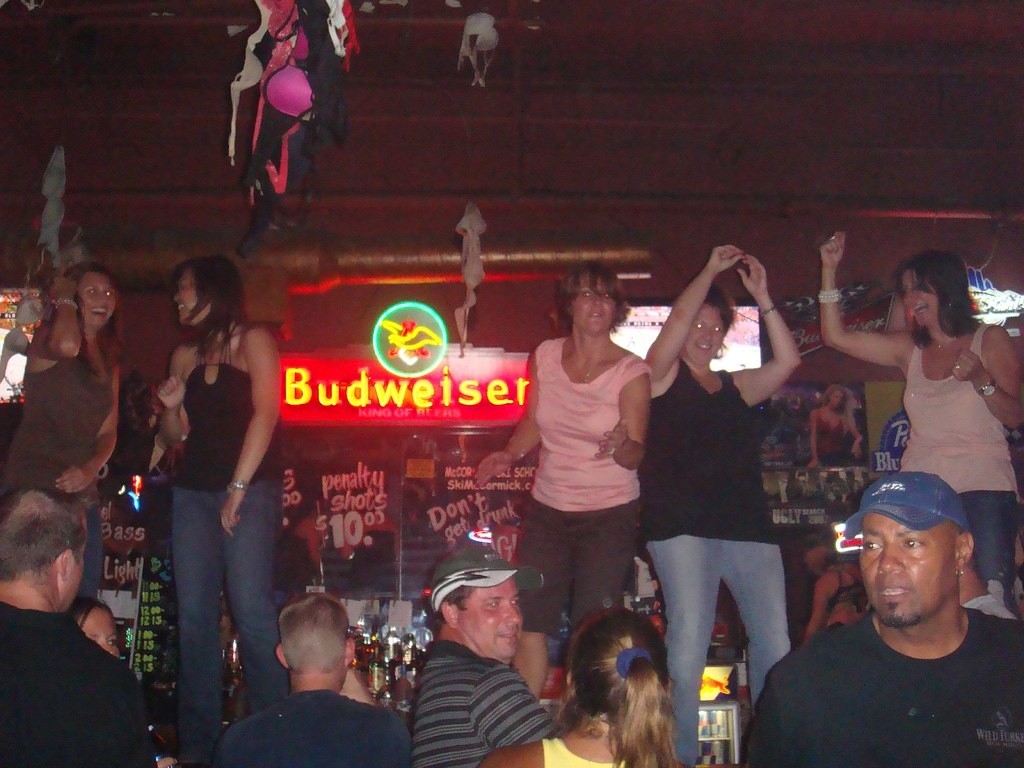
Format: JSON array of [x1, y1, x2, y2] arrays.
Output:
[[345, 613, 424, 713], [557, 612, 571, 667], [221, 632, 243, 677]]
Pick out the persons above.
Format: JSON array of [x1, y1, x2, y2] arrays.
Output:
[[210, 592, 413, 768], [0, 261, 121, 601], [817, 231, 1024, 621], [412, 550, 564, 768], [154, 255, 291, 768], [802, 482, 1017, 642], [0, 484, 178, 768], [641, 246, 802, 768], [746, 471, 1024, 768], [808, 384, 863, 468], [475, 261, 652, 702], [473, 608, 687, 768]]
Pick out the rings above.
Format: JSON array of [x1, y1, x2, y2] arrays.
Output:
[[609, 448, 614, 454], [955, 365, 960, 369], [831, 236, 835, 239]]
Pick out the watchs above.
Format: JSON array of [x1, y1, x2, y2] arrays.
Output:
[[977, 378, 995, 396]]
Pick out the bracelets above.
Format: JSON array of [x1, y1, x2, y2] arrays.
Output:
[[762, 306, 775, 315], [55, 299, 77, 310], [818, 289, 840, 303], [229, 481, 248, 490]]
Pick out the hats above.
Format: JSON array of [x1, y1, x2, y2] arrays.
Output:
[[843, 471, 973, 540], [430, 544, 544, 615]]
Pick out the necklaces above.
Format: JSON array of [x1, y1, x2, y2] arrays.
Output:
[[582, 370, 592, 383]]
